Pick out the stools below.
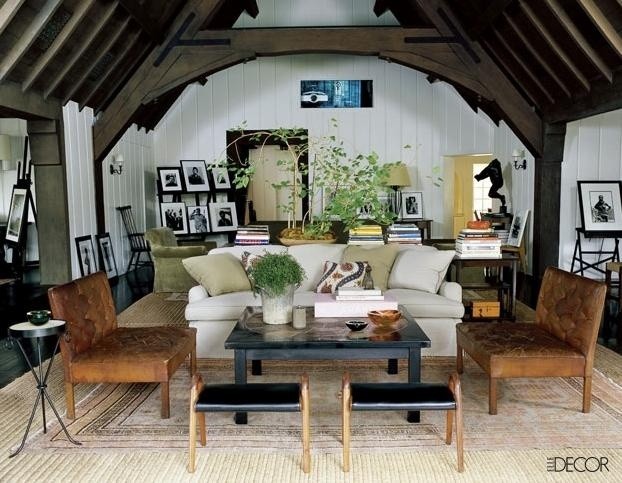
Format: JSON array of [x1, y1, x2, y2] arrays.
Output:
[[606, 261, 620, 297], [189, 372, 311, 474], [341, 370, 464, 472]]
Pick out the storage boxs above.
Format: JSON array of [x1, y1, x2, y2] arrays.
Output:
[[470, 298, 500, 318]]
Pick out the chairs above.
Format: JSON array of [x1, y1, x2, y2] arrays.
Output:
[[455, 264, 607, 414], [48, 271, 196, 418], [145, 225, 217, 294], [117, 205, 155, 275]]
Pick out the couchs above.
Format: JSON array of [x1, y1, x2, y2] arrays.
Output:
[[187, 244, 466, 358]]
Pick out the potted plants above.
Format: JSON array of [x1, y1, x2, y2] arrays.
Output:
[[248, 247, 307, 325], [210, 120, 397, 246]]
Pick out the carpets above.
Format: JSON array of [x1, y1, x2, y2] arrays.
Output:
[[12, 352, 622, 450]]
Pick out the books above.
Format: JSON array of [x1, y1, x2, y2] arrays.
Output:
[[335, 286, 384, 300], [235, 225, 270, 246], [346, 225, 422, 246], [455, 229, 509, 259]]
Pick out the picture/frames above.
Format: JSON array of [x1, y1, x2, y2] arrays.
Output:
[[4, 184, 29, 245], [508, 209, 530, 249], [75, 232, 119, 286], [355, 196, 377, 218], [577, 180, 621, 233], [401, 191, 425, 219], [157, 158, 238, 236]]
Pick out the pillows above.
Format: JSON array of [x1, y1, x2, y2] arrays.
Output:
[[181, 253, 252, 296], [315, 244, 455, 293]]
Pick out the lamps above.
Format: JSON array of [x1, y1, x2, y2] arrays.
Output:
[[110, 155, 123, 175], [513, 149, 526, 170], [382, 163, 412, 219]]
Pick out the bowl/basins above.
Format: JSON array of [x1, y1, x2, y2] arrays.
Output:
[[27, 310, 52, 326], [345, 320, 369, 331], [367, 310, 402, 327]]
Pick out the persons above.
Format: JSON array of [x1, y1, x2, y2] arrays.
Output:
[[594, 195, 612, 222], [165, 208, 183, 229], [474, 158, 506, 206], [218, 211, 233, 226], [166, 175, 178, 185], [189, 208, 206, 232], [84, 247, 93, 274], [102, 241, 112, 271], [188, 167, 204, 185], [406, 197, 417, 215]]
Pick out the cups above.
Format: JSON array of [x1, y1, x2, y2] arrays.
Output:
[[292, 305, 306, 329]]
